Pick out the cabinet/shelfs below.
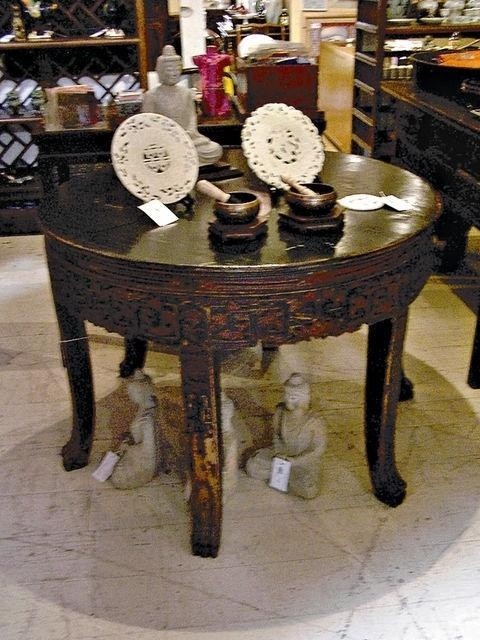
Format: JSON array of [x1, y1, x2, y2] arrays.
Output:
[[351, 0, 479, 156], [0, 1, 142, 235]]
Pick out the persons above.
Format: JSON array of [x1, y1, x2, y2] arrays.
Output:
[[183, 391, 239, 503], [107, 372, 163, 489], [142, 46, 222, 171], [245, 374, 328, 500]]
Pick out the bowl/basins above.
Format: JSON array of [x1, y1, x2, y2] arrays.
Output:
[[288, 182, 337, 216], [420, 18, 444, 25], [387, 18, 417, 27], [213, 190, 261, 224]]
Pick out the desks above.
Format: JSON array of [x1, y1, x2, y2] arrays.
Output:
[[32, 144, 445, 556]]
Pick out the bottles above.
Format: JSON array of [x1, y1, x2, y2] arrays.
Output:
[[279, 8, 289, 27]]
